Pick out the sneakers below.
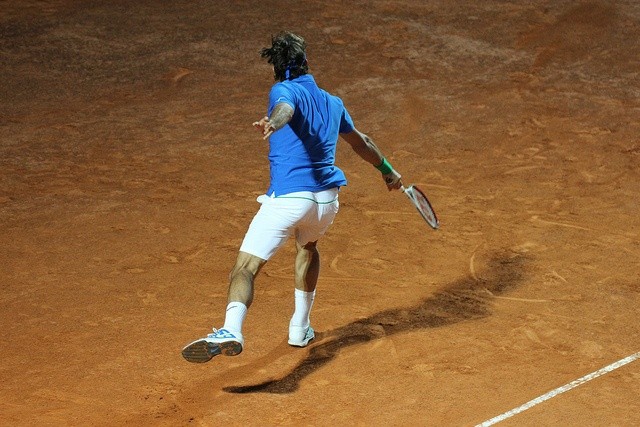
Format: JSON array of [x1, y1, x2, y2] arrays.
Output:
[[180, 327, 244, 364], [288, 314, 315, 347]]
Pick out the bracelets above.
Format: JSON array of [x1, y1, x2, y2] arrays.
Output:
[[375, 157, 394, 176]]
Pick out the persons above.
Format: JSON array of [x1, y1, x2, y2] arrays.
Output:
[[182, 29, 402, 363]]
